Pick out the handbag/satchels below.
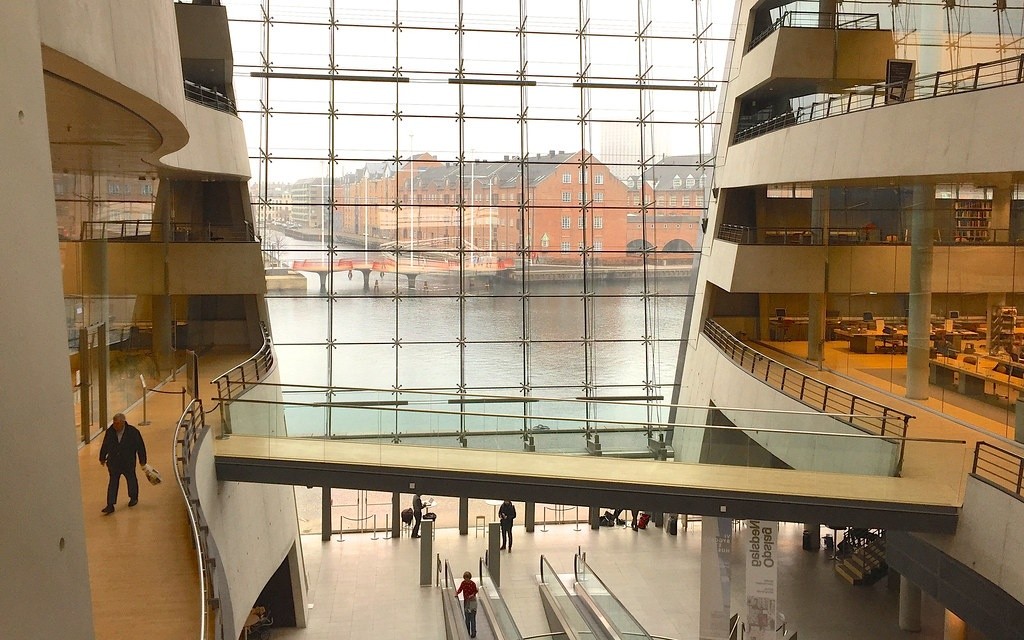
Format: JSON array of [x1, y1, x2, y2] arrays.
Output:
[[638, 513, 650, 530], [143, 465, 163, 486]]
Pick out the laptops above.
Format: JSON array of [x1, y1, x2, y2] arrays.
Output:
[[863, 312, 874, 322]]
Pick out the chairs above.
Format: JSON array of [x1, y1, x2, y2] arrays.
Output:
[[953, 357, 979, 381], [208, 230, 224, 241], [777, 320, 793, 342], [983, 365, 1009, 400], [892, 333, 904, 354]]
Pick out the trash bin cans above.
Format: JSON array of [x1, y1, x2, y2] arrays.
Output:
[[803, 530, 810, 550], [1013, 397, 1024, 446]]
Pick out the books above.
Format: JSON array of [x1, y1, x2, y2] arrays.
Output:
[[954, 200, 993, 243]]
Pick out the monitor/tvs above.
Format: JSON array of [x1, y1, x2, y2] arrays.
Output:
[[949, 311, 959, 319], [775, 308, 787, 317]]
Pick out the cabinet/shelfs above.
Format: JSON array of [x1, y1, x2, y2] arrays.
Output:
[[954, 201, 993, 243]]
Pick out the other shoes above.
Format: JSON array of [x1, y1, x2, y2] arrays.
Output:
[[471, 633, 477, 638], [631, 524, 639, 532], [128, 496, 138, 507], [412, 534, 421, 538], [508, 547, 511, 553], [500, 545, 506, 550], [102, 505, 115, 513]]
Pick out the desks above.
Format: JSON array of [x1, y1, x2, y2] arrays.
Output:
[[769, 318, 1024, 405], [243, 613, 260, 640]]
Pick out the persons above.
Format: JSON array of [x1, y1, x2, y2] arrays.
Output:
[[499, 499, 516, 553], [98, 412, 150, 514], [455, 571, 479, 638], [410, 495, 427, 539]]
